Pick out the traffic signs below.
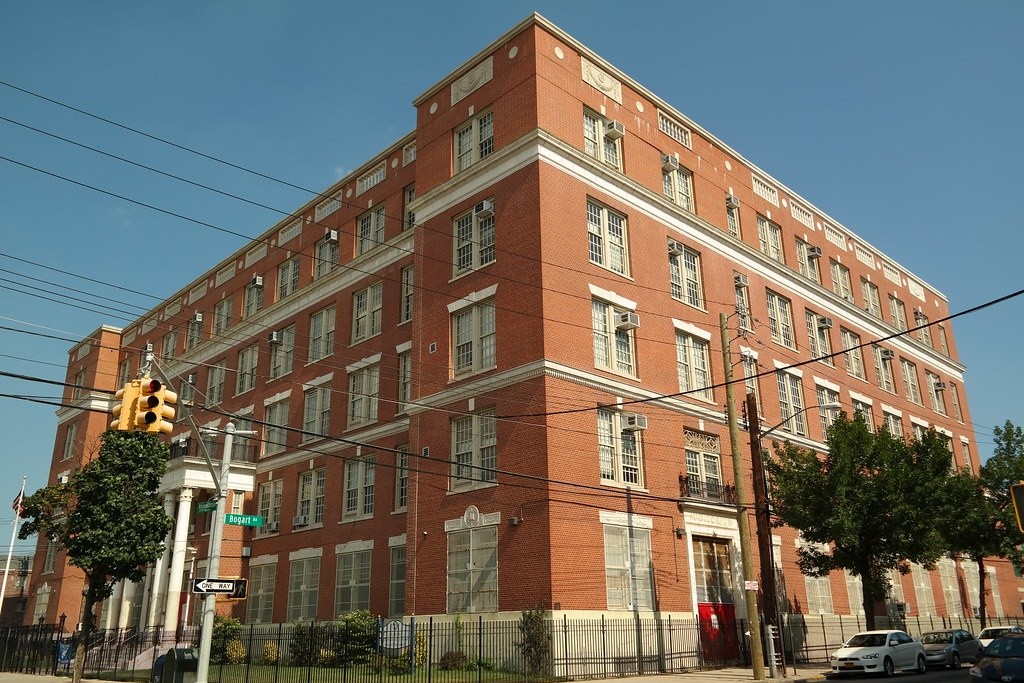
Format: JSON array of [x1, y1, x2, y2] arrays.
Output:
[[192, 577, 237, 595]]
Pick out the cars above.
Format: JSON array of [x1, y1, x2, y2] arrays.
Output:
[[977, 626, 1024, 654], [830, 629, 928, 678], [968, 632, 1024, 683], [918, 628, 984, 671]]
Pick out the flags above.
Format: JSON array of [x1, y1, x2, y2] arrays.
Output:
[[13, 489, 24, 514]]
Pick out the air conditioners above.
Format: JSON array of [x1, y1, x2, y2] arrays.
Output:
[[667, 245, 946, 391], [474, 200, 493, 218], [896, 602, 910, 614], [615, 312, 640, 332], [726, 195, 740, 209], [143, 230, 340, 385], [605, 120, 625, 140], [188, 524, 194, 534], [662, 154, 679, 174], [974, 607, 988, 618], [622, 413, 648, 431], [266, 515, 309, 532]]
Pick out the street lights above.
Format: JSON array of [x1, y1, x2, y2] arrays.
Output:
[[747, 400, 845, 679]]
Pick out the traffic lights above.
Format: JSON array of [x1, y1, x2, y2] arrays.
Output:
[[133, 378, 161, 429], [227, 579, 249, 600], [147, 385, 177, 436], [110, 382, 140, 431]]
